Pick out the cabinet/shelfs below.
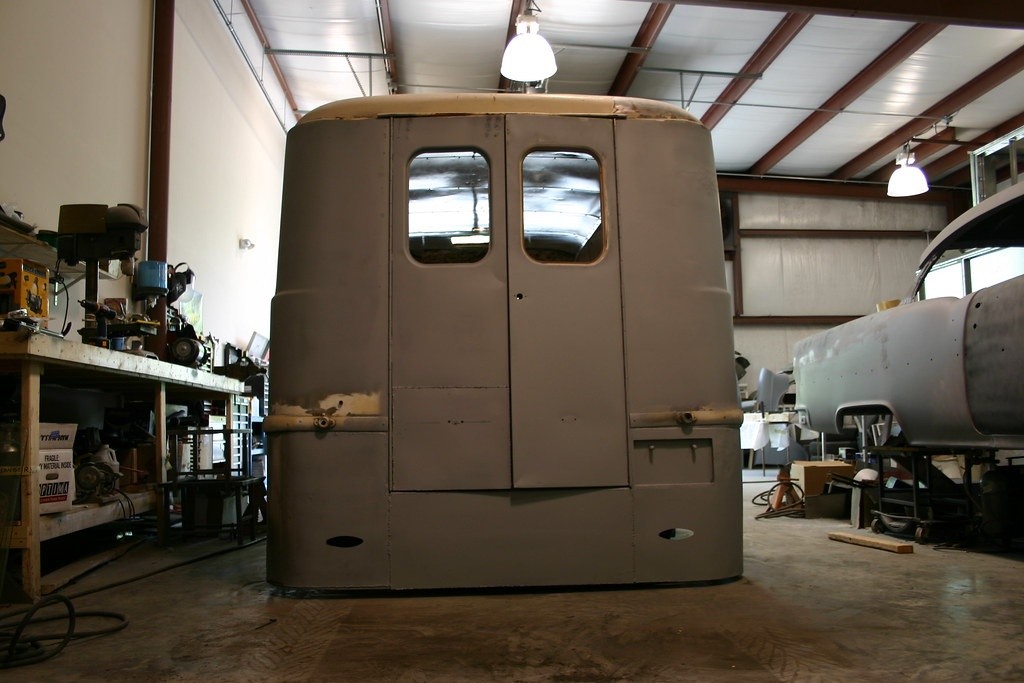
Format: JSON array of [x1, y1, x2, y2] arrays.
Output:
[[865, 447, 995, 524], [0, 331, 245, 604], [170, 428, 253, 479]]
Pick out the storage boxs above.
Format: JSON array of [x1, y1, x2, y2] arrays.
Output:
[[789, 460, 855, 508], [39, 423, 78, 514]]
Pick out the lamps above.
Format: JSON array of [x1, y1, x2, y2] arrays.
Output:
[[887, 142, 930, 198], [500, 0, 558, 82]]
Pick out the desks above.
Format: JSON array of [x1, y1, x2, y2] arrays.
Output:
[[156, 476, 266, 546]]
